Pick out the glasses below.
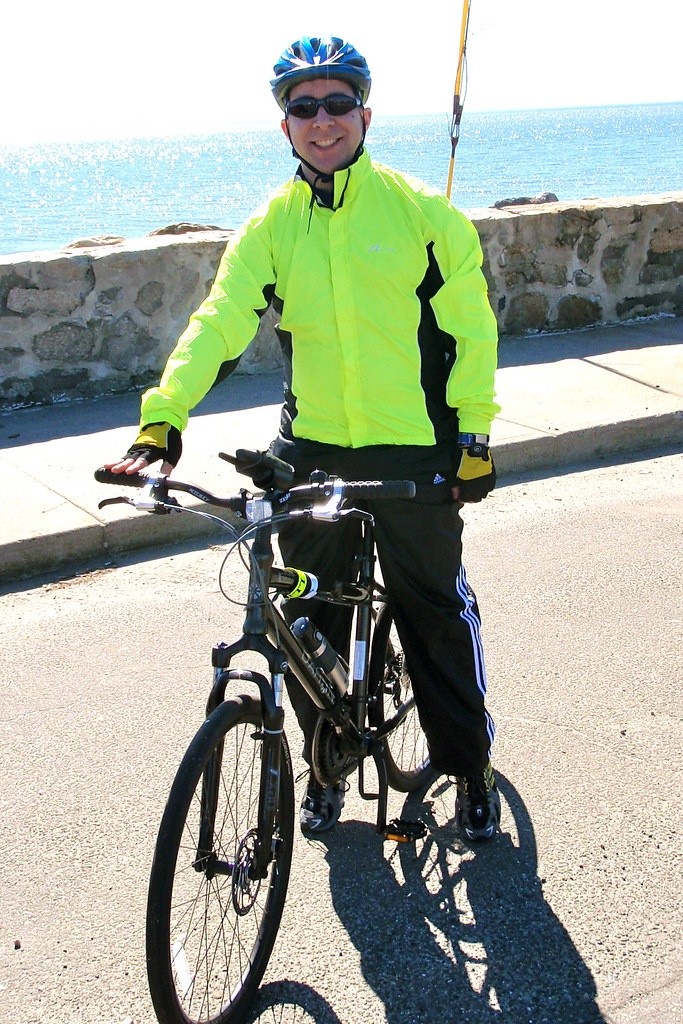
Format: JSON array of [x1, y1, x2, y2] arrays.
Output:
[[285, 93, 361, 120]]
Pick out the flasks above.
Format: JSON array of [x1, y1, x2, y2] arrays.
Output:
[[289, 617, 350, 697]]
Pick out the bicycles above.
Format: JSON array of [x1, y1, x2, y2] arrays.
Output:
[[97, 447, 435, 1023]]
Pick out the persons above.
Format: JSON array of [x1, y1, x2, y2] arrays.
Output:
[[103, 34, 501, 849]]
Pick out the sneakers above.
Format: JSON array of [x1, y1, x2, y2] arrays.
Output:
[[447, 756, 501, 848], [295, 765, 351, 834]]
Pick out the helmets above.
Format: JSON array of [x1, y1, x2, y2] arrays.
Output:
[[269, 35, 372, 115]]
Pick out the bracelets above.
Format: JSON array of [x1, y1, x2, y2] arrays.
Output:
[[458, 433, 489, 446]]
